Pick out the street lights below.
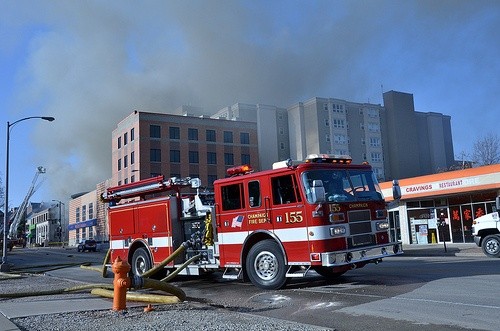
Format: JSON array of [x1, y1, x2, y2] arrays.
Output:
[[2, 116, 55, 266]]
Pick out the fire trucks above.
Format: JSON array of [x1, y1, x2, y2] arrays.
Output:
[[100, 153, 404, 292]]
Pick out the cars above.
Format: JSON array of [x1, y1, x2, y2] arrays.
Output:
[[77, 238, 97, 253], [472, 194, 500, 258]]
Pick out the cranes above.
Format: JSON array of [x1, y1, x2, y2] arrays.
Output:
[[8, 167, 47, 238]]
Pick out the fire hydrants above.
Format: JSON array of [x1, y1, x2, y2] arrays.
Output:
[[109, 255, 132, 312]]
